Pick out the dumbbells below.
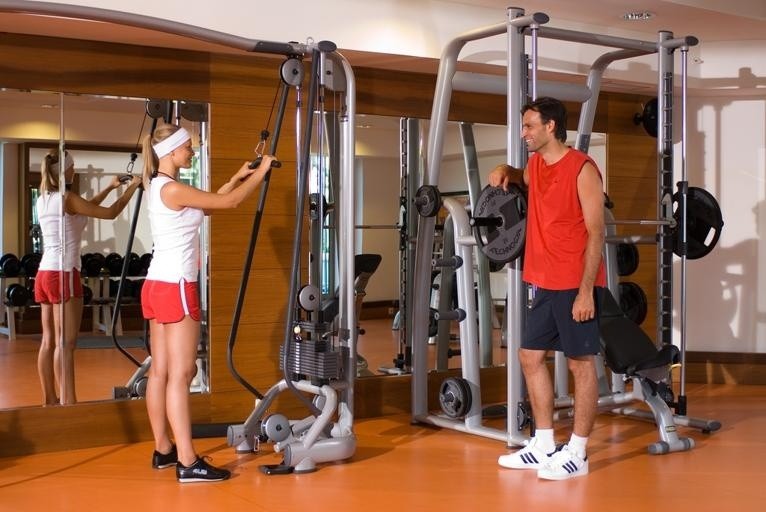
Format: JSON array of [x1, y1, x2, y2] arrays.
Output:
[[0, 252, 153, 307]]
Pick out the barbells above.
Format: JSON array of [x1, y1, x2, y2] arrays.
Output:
[[470, 183, 724, 265]]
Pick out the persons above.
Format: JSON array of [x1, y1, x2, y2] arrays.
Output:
[[140, 124, 278, 484], [34, 149, 143, 405], [486, 95, 605, 481]]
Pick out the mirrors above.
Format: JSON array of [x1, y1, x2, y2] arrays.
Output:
[[310, 111, 608, 378], [0, 87, 212, 413]]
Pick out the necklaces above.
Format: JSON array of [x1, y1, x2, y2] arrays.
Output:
[[157, 172, 175, 180]]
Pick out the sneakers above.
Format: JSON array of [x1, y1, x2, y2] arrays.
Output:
[[498, 438, 561, 469], [536, 442, 589, 480], [176, 454, 231, 482], [152, 444, 178, 468]]
